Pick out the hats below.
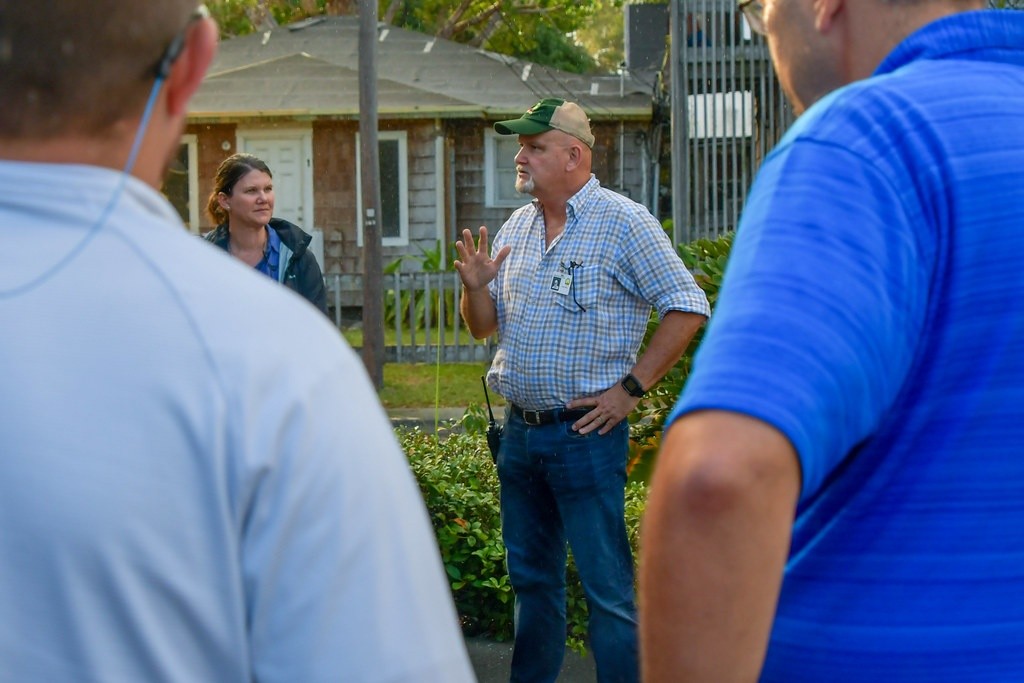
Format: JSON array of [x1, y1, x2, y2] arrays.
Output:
[[494, 98, 595, 148]]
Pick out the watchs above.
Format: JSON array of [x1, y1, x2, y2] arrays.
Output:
[[621, 374, 646, 397]]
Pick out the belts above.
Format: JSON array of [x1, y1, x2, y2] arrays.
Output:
[[508, 405, 597, 426]]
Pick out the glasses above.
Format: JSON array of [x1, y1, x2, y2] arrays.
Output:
[[736, 0, 769, 36]]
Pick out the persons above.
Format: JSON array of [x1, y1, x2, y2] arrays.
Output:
[[636, 0, 1024, 683], [202, 152, 327, 315], [0, 0, 480, 683], [453, 97, 712, 683]]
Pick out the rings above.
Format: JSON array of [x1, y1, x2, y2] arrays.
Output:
[[597, 417, 604, 423]]
